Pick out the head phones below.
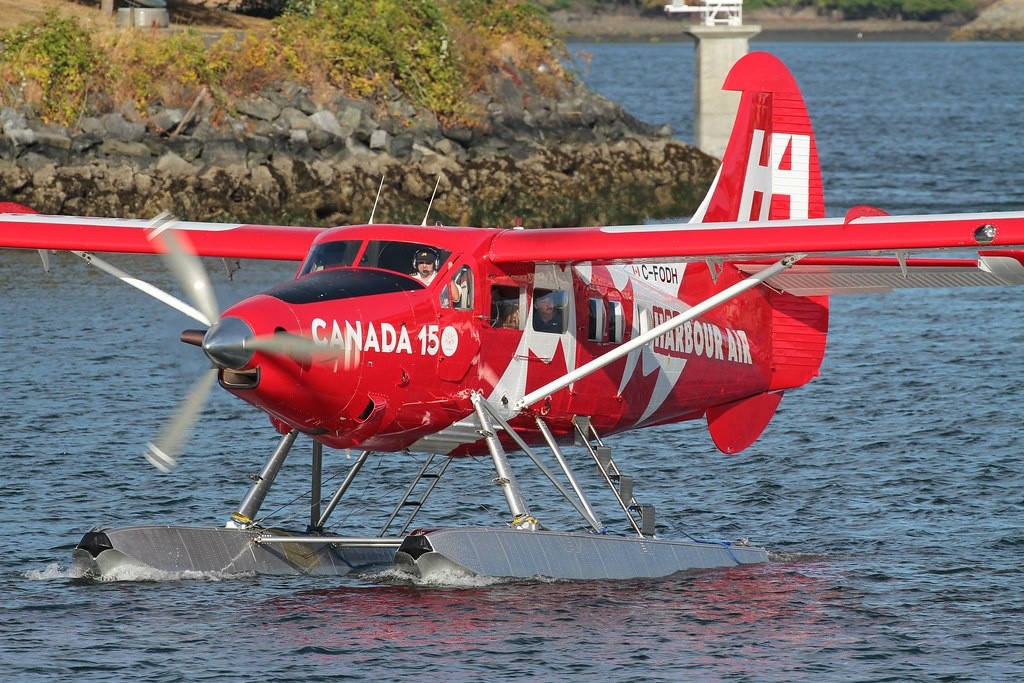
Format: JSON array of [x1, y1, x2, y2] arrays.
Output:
[[412, 247, 440, 270]]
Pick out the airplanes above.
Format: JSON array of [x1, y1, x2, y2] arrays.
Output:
[[1, 48, 1024, 586]]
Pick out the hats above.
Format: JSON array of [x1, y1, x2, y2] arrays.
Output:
[[416, 248, 437, 264]]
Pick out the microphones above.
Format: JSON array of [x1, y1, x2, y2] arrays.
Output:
[[422, 269, 437, 274]]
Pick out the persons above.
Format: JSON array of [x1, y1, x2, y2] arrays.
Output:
[[409, 247, 463, 309], [532, 288, 564, 335]]
